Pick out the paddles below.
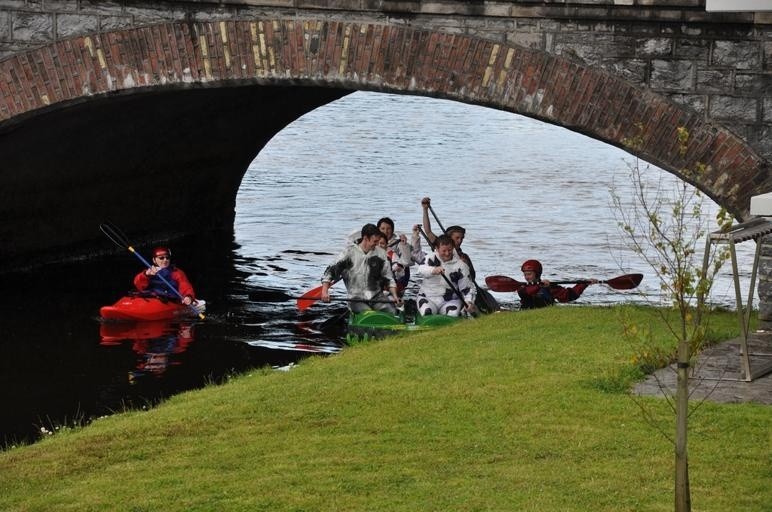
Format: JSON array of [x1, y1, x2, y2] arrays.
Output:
[[322, 289, 384, 327], [298, 240, 403, 310], [99, 218, 205, 321], [485, 276, 643, 292], [426, 201, 500, 314], [249, 290, 400, 303]]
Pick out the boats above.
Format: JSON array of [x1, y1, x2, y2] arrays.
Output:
[[343, 299, 455, 343], [98, 317, 174, 347], [98, 290, 209, 320]]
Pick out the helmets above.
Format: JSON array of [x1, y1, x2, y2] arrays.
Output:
[[521, 260, 542, 274], [152, 247, 172, 258]]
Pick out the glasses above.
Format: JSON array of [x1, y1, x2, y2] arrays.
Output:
[[159, 257, 170, 259]]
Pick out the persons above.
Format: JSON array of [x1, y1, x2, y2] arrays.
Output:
[[133, 247, 195, 305], [320, 197, 479, 318], [517, 260, 599, 309]]
